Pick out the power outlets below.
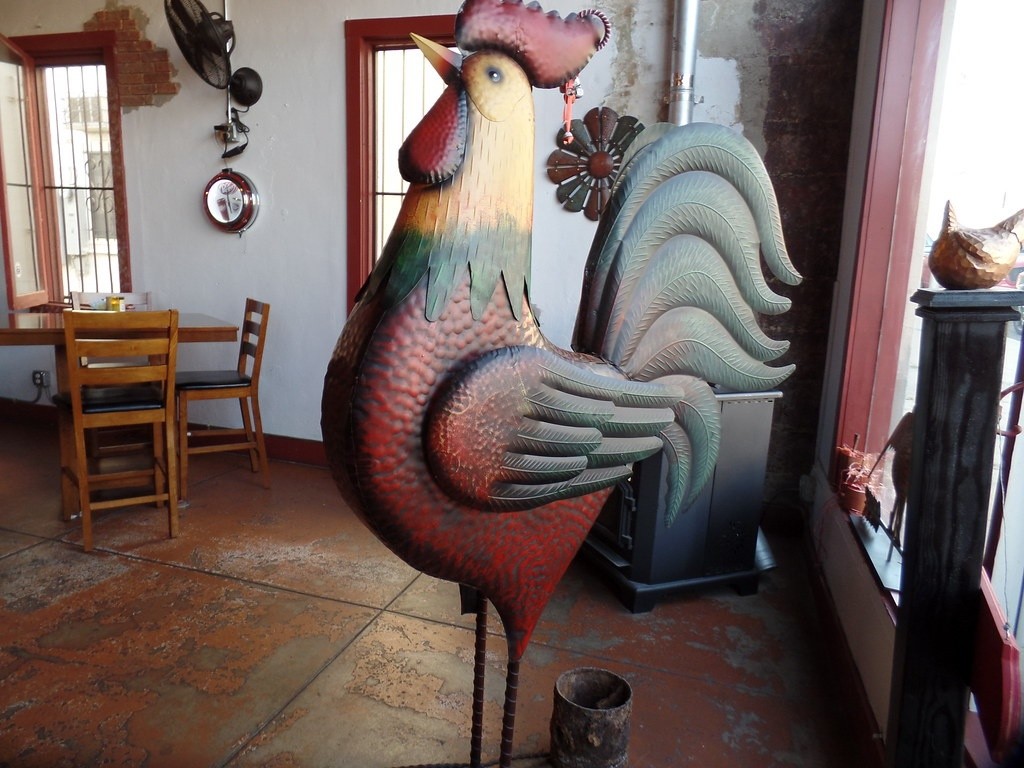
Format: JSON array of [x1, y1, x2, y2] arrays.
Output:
[[32, 370, 50, 387]]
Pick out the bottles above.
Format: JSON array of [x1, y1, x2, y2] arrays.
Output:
[[126, 304, 135, 311], [119, 297, 125, 312]]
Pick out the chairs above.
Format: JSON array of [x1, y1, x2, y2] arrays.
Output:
[[175, 296, 272, 503], [51, 309, 179, 552]]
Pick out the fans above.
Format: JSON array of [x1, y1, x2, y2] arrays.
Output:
[[163, 0, 263, 107]]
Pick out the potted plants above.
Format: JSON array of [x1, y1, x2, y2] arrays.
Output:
[[843, 463, 873, 515]]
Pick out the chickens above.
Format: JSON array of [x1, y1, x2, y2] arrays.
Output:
[[321, 0, 803, 768]]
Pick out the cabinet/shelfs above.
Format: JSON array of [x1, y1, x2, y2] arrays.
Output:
[[576, 388, 779, 615]]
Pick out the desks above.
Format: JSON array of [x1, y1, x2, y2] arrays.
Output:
[[0, 312, 240, 492]]
